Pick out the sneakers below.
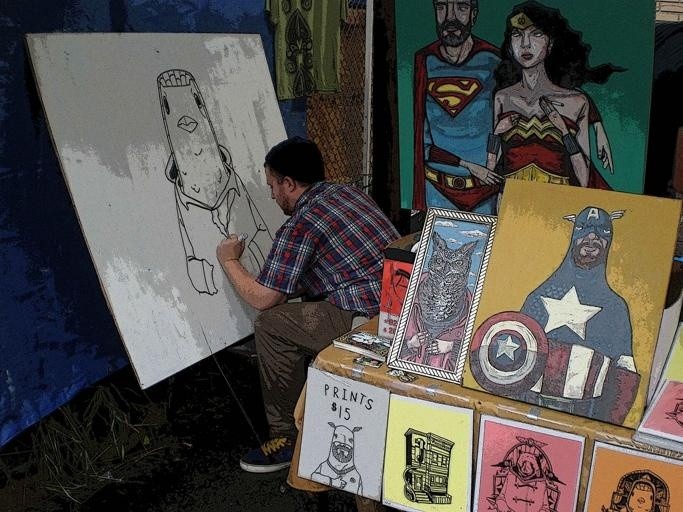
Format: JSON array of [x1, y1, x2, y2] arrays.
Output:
[[239, 435, 298, 473]]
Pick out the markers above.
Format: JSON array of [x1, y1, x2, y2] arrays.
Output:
[[238, 232, 248, 242]]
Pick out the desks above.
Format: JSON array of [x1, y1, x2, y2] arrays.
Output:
[[286, 314, 683, 512]]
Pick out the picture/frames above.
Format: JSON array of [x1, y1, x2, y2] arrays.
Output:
[[388, 207, 501, 385]]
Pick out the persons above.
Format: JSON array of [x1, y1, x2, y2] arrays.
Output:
[[215, 134, 403, 474]]
